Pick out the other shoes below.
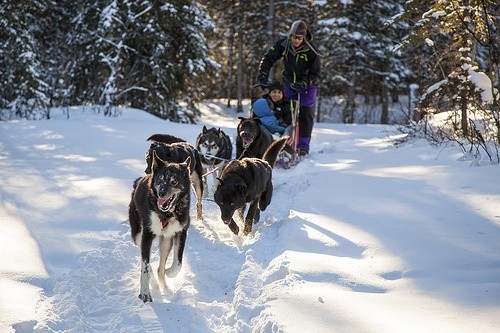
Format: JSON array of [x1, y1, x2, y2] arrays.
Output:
[[300, 151, 306, 156]]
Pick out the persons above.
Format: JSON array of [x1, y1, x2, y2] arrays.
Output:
[[257, 21, 322, 161], [253, 81, 294, 145]]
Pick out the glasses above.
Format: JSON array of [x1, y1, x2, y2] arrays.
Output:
[[293, 35, 303, 39]]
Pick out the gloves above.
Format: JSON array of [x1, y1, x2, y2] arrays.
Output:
[[291, 80, 307, 91], [258, 73, 268, 90]]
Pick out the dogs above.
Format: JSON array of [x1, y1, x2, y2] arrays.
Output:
[[195, 125, 233, 199], [213, 137, 288, 236], [236, 117, 273, 158], [128, 134, 204, 303]]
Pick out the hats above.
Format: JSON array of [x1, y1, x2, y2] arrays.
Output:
[[268, 79, 285, 90], [291, 19, 308, 34]]
